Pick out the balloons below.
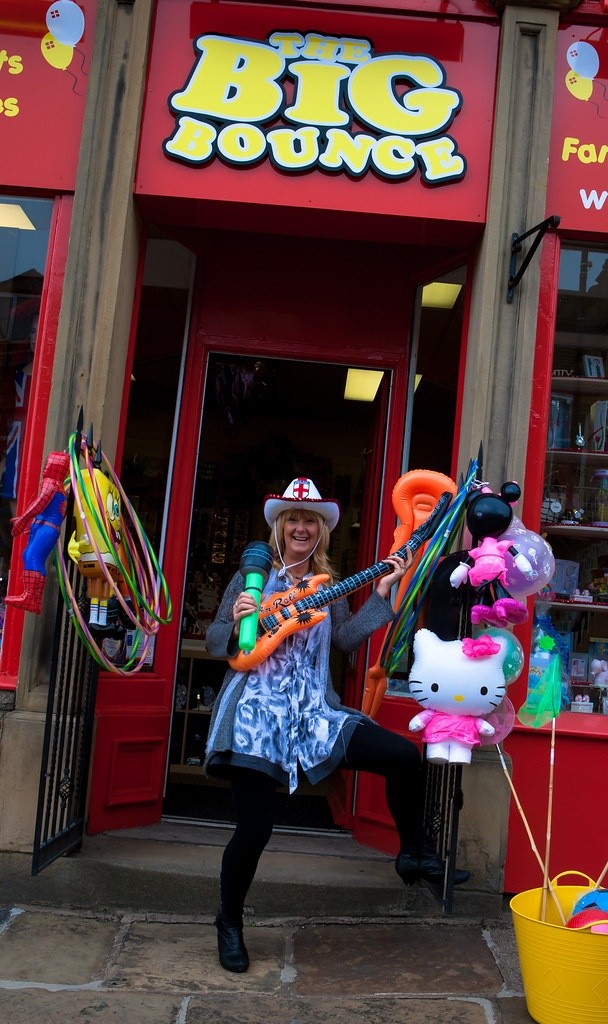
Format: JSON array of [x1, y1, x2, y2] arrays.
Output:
[[476, 694, 516, 745], [472, 626, 525, 687], [490, 529, 555, 597]]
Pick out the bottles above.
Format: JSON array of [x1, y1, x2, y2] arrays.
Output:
[[589, 469, 608, 527]]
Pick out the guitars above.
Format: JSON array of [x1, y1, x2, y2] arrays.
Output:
[[226, 492, 454, 672], [361, 469, 459, 719]]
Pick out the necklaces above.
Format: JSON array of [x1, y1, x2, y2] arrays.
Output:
[[294, 576, 304, 581]]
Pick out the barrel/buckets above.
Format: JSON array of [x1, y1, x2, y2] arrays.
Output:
[[509, 871, 608, 1024]]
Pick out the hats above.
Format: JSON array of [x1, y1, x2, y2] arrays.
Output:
[[265, 478, 341, 533]]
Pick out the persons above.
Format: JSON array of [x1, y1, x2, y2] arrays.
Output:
[[4, 451, 71, 615], [203, 478, 471, 973]]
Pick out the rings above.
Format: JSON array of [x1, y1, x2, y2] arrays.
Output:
[[400, 564, 405, 568], [235, 600, 239, 608]]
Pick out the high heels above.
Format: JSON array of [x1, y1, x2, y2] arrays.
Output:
[[393, 854, 471, 886], [212, 908, 251, 973]]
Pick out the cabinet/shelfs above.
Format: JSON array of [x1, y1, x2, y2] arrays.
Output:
[[170, 639, 327, 791], [534, 375, 608, 715]]
